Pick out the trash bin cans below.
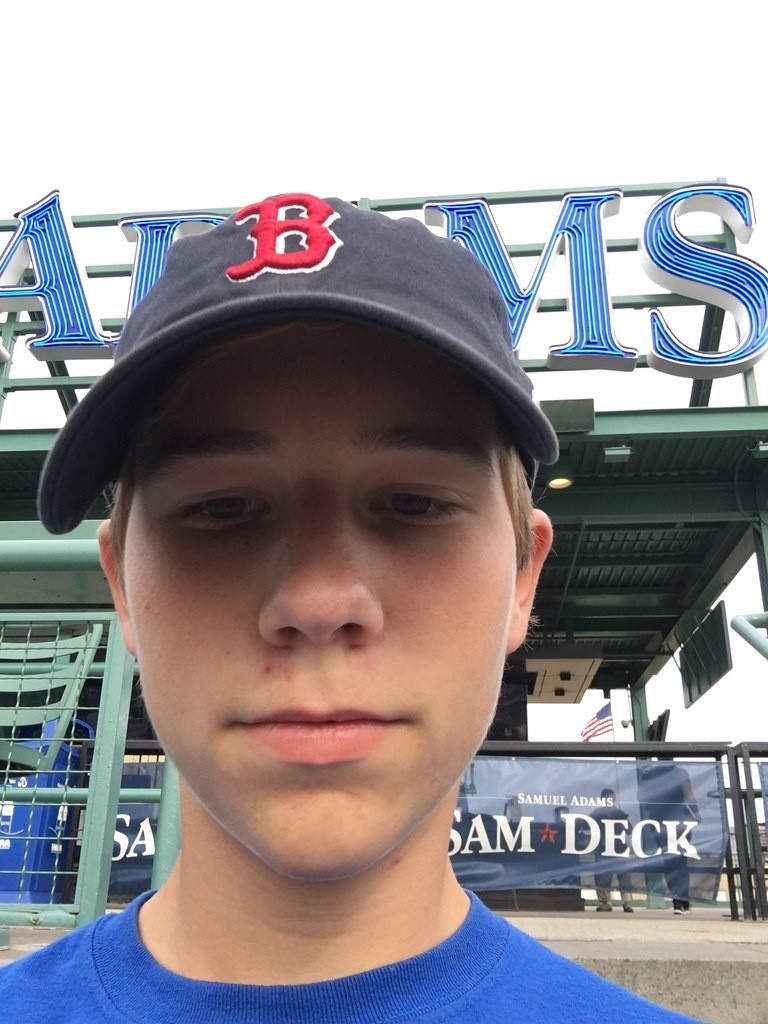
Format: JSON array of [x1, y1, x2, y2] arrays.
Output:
[[0, 712, 96, 904]]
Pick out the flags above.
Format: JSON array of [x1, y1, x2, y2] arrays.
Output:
[[580, 702, 613, 743]]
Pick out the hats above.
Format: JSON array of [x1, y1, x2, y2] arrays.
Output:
[[38, 193, 558, 534]]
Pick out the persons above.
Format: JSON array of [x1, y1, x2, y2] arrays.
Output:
[[1, 197, 720, 1021], [638, 754, 700, 915], [585, 786, 637, 913]]
